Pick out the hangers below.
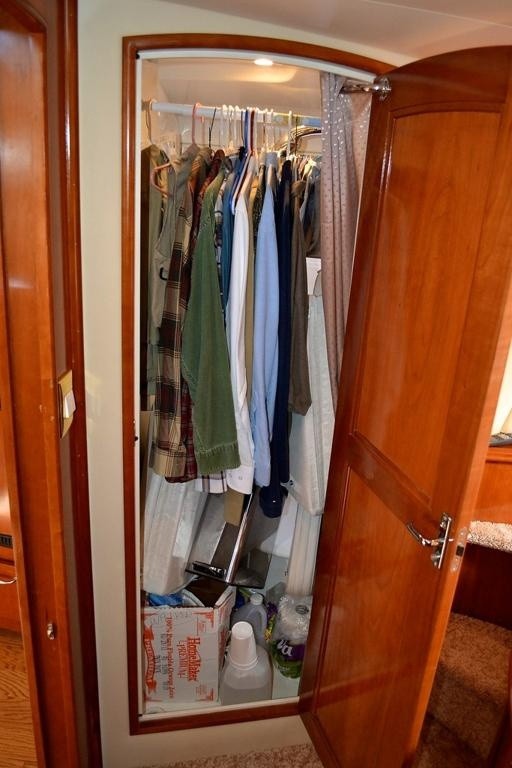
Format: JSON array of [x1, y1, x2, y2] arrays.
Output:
[[141, 96, 328, 214]]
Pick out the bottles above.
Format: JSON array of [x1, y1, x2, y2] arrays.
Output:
[[270, 609, 278, 635], [270, 605, 311, 679]]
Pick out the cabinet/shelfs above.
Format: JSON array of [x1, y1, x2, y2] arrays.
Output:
[[114, 25, 510, 763]]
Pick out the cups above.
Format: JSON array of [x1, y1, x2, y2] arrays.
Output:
[[225, 621, 259, 668]]
